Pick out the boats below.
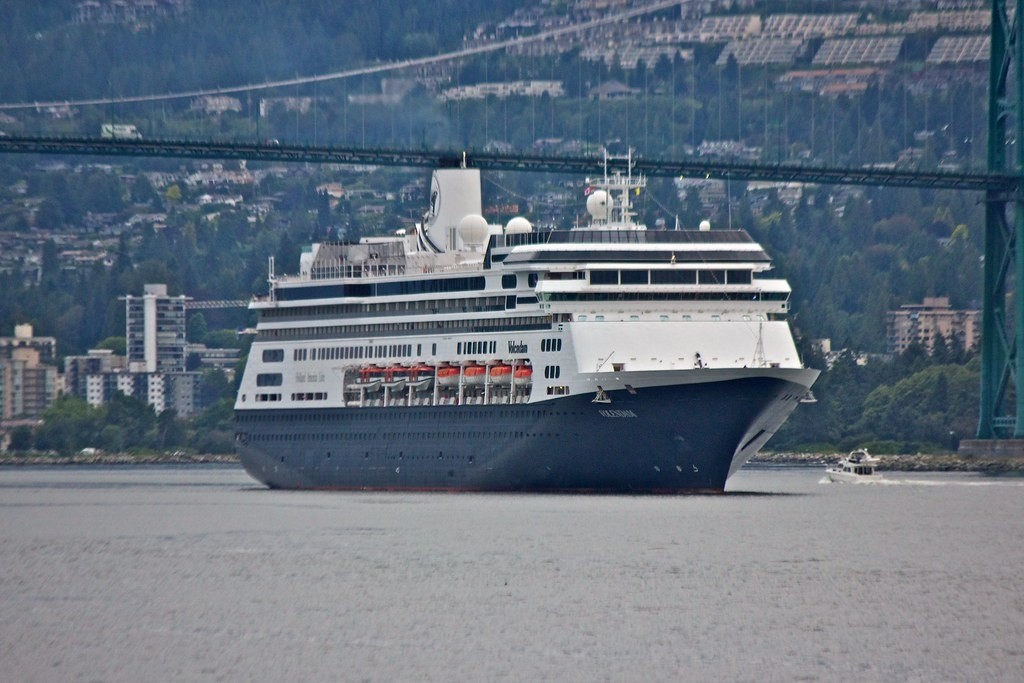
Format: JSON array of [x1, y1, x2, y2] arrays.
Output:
[[229, 149, 821, 496], [826, 448, 883, 485]]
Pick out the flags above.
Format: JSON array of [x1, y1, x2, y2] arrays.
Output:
[[583, 185, 594, 197]]
